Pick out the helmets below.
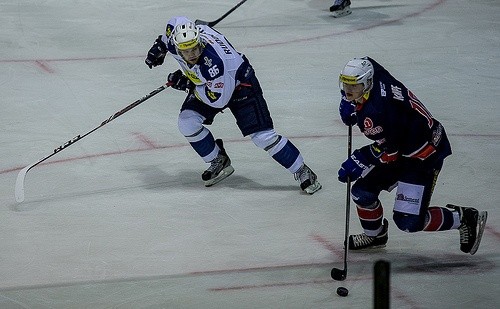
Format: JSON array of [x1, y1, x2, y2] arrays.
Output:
[[338, 57, 374, 93], [171, 22, 201, 55]]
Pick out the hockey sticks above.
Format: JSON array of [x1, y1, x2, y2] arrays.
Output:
[[331, 119, 354, 282], [194, 0, 246, 26], [14, 82, 176, 205]]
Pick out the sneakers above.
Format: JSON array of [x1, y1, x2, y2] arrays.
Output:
[[330, 0, 352, 18], [446, 203, 488, 255], [201, 138, 235, 187], [344, 217, 389, 251], [295, 163, 322, 195]]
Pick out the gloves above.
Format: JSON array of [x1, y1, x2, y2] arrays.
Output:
[[167, 69, 197, 95], [338, 149, 373, 183], [145, 34, 169, 69], [338, 94, 358, 126]]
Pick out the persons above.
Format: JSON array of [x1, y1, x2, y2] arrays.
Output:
[[330, 0, 352, 18], [144, 16, 322, 195], [337, 55, 488, 256]]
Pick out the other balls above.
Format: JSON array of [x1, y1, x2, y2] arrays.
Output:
[[337, 286, 349, 297]]
